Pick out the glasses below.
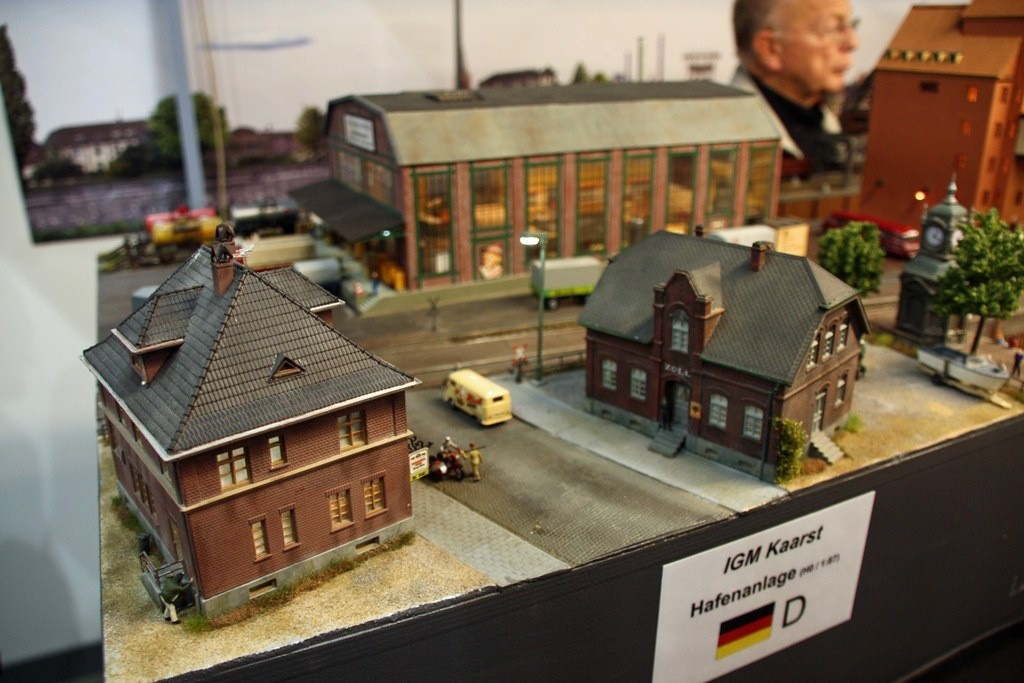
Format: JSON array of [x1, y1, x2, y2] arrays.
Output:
[[760, 18, 860, 40]]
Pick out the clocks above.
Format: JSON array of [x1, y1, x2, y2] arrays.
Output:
[[925, 225, 944, 247], [952, 229, 964, 246]]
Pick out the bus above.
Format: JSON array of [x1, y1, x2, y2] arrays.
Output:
[[822, 210, 920, 258], [445, 369, 512, 425]]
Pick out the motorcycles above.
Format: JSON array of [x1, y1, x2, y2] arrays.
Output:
[[429, 449, 464, 480]]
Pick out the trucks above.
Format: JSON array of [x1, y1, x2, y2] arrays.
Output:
[[531, 255, 600, 311]]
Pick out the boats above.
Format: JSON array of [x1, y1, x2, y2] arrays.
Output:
[[915, 339, 1010, 394]]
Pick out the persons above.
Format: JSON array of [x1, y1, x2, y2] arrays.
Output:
[[439, 436, 461, 459], [372, 271, 379, 294], [730, 0, 859, 179], [1011, 347, 1024, 377], [460, 442, 484, 482]]
